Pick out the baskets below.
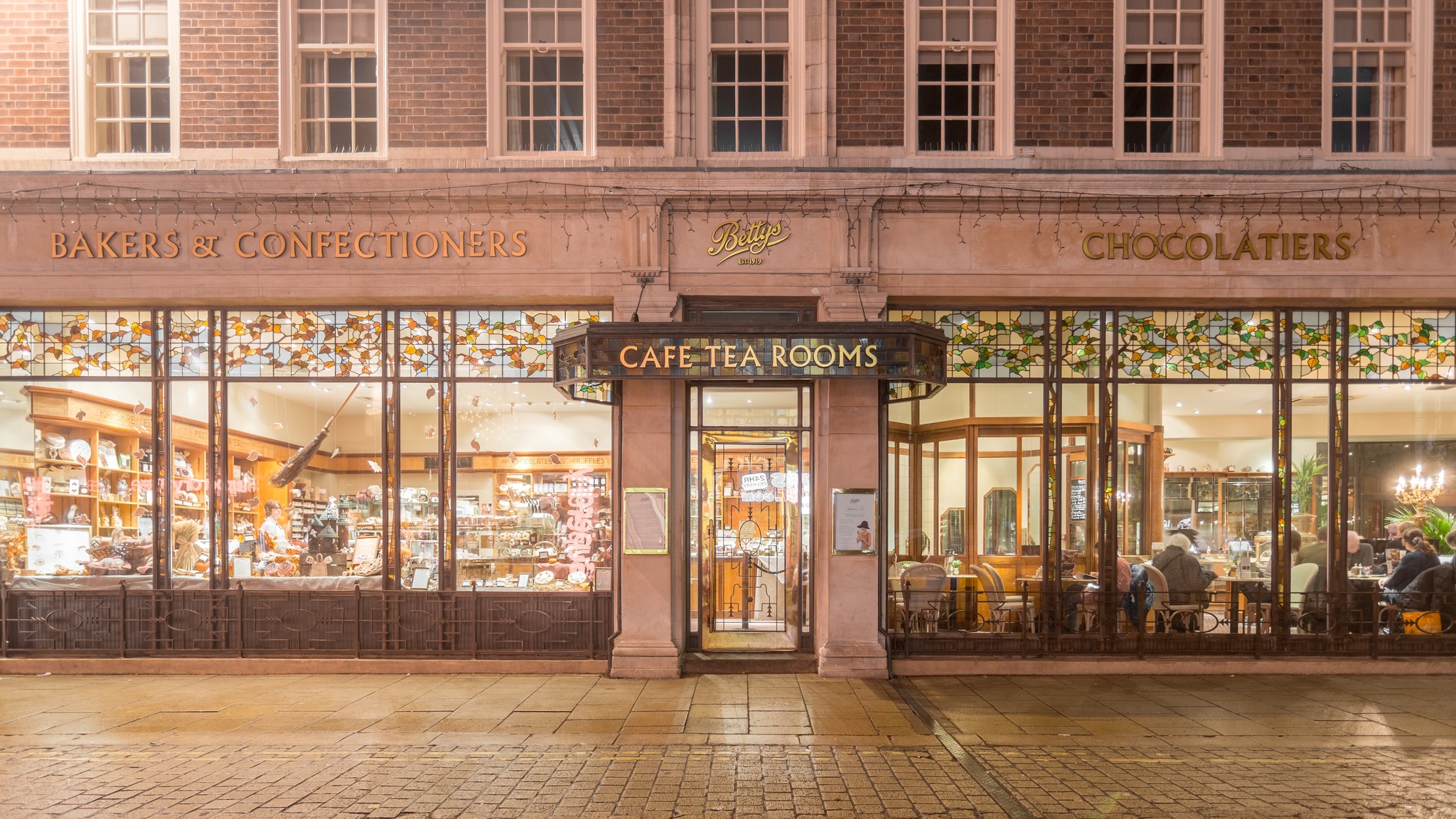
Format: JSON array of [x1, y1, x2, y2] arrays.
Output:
[[589, 561, 604, 567], [142, 556, 153, 575], [159, 557, 165, 574], [531, 541, 558, 563], [125, 560, 141, 575], [83, 556, 126, 575], [112, 529, 152, 560], [86, 536, 118, 561], [558, 542, 583, 563]]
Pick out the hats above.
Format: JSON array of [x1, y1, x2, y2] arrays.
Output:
[[857, 521, 870, 529]]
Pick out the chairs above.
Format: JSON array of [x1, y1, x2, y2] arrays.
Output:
[[1377, 562, 1453, 634], [894, 562, 948, 634], [887, 560, 936, 632], [1075, 559, 1153, 633], [978, 560, 1036, 633], [1138, 563, 1205, 633], [1246, 562, 1319, 634], [968, 564, 1034, 634]]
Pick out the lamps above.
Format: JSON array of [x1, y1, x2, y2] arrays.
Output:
[[1394, 462, 1446, 539]]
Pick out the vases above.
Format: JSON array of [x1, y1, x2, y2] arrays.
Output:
[[954, 567, 959, 575]]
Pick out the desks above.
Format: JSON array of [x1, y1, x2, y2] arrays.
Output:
[[886, 572, 979, 630], [1346, 575, 1395, 634], [1015, 577, 1098, 631], [1208, 576, 1273, 632]]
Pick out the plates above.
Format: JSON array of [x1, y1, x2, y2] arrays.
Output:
[[1032, 577, 1042, 579]]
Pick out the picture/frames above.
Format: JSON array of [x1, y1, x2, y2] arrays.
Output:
[[517, 571, 531, 588], [410, 565, 433, 590], [352, 535, 381, 565]]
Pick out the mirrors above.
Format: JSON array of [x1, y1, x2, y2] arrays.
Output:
[[940, 507, 966, 555], [906, 531, 930, 555], [984, 486, 1017, 556], [1026, 463, 1041, 545]]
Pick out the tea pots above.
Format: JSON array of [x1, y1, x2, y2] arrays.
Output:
[[1036, 566, 1043, 577], [1351, 564, 1369, 576]]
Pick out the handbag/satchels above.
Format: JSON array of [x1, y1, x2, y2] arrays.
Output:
[[311, 553, 327, 577]]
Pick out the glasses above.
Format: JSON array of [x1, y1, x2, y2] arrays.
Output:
[[272, 506, 282, 511]]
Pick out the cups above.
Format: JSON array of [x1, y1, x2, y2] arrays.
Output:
[[1177, 466, 1183, 471]]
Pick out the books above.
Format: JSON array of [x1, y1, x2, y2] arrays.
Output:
[[1073, 572, 1097, 580]]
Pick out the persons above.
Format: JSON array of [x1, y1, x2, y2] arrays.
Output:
[[261, 500, 302, 554], [541, 492, 610, 537], [1262, 527, 1456, 635], [857, 521, 871, 549], [1031, 541, 1132, 633], [1151, 533, 1218, 633]]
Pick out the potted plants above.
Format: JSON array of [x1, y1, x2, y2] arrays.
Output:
[[1290, 451, 1329, 536]]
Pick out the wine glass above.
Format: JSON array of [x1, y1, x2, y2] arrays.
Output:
[[1223, 562, 1231, 578]]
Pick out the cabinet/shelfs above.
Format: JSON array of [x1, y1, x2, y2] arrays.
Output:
[[354, 472, 612, 587], [292, 496, 336, 541], [1162, 477, 1306, 557], [0, 406, 258, 584], [690, 468, 786, 553]]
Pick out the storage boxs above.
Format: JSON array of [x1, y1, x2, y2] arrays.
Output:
[[298, 552, 353, 577], [293, 477, 327, 538]]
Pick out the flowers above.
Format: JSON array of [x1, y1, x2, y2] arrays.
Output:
[[901, 562, 911, 569], [953, 558, 963, 568]]
